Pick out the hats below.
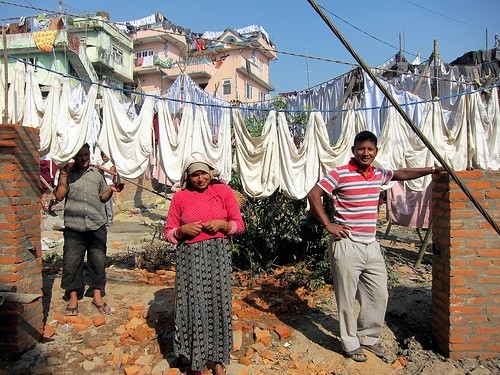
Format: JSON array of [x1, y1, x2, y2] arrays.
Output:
[[186, 162, 211, 176]]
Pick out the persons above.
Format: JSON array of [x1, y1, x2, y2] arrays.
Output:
[[98, 151, 117, 225], [163, 152, 245, 375], [306, 131, 448, 362], [53, 141, 125, 315]]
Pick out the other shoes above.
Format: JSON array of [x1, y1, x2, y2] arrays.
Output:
[[105, 220, 113, 224], [194, 366, 213, 375]]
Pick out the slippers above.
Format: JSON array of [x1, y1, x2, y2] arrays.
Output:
[[345, 347, 368, 361], [360, 343, 389, 356], [65, 302, 79, 316], [92, 301, 111, 315]]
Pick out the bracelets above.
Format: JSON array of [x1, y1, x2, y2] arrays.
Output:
[[431, 166, 435, 173]]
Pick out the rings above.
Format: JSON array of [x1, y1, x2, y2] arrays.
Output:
[[340, 230, 343, 234]]
